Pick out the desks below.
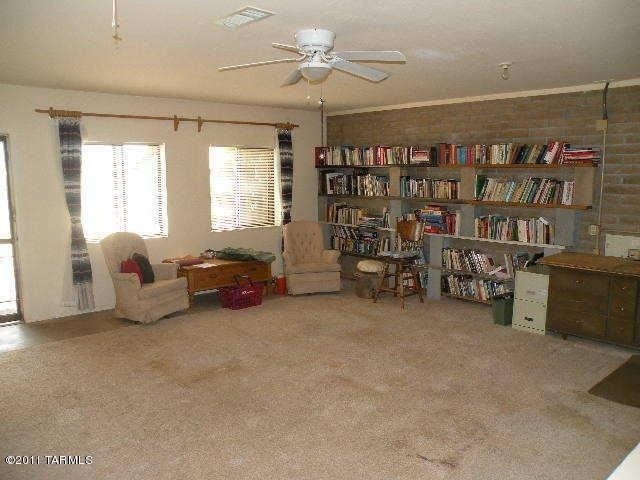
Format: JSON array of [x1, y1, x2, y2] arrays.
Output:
[[162, 256, 271, 311]]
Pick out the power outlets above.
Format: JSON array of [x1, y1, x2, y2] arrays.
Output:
[[589, 224, 598, 236]]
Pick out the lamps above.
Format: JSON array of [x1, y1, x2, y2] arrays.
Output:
[[299, 62, 332, 83]]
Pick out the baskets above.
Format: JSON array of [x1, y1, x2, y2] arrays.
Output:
[[217, 274, 264, 310]]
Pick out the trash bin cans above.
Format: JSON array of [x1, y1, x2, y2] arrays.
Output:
[[492, 292, 514, 326]]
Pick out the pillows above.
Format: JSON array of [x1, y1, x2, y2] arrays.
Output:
[[120, 253, 155, 283]]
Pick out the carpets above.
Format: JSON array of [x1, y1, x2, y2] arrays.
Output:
[[587, 353, 640, 408]]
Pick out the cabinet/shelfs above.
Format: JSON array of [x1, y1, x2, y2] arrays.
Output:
[[315, 161, 599, 305], [513, 251, 640, 351]]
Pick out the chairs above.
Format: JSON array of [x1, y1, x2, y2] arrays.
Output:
[[281, 221, 341, 297], [604, 234, 640, 258], [98, 230, 190, 324], [373, 220, 424, 312]]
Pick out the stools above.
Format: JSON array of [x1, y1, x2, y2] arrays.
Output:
[[355, 259, 384, 299]]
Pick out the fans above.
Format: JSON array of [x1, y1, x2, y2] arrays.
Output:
[[218, 29, 409, 88]]
